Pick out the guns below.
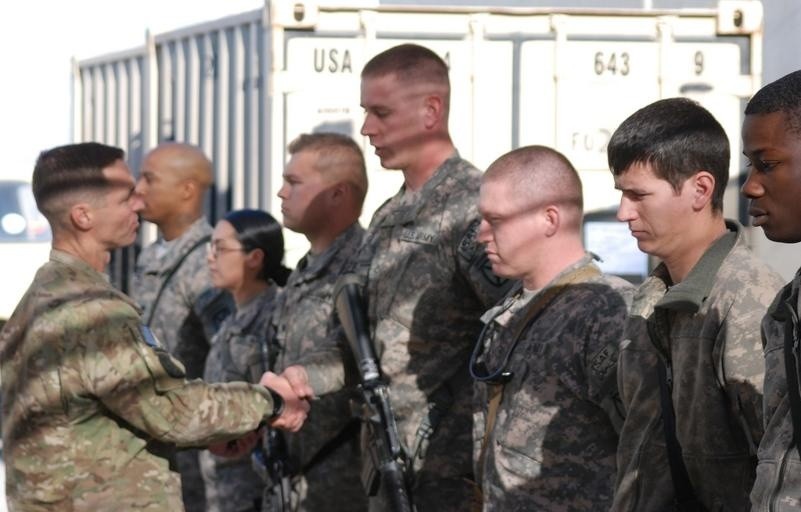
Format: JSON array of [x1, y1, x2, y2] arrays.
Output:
[[247, 341, 292, 512], [333, 271, 412, 511]]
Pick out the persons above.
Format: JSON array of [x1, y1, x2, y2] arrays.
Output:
[[270, 45, 521, 512], [253, 133, 369, 512], [607, 99, 786, 512], [0, 144, 311, 512], [471, 146, 638, 512], [198, 211, 293, 512], [739, 71, 801, 512], [128, 143, 234, 512]]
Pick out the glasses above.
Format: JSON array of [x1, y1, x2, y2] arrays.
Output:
[[205, 241, 245, 255]]
